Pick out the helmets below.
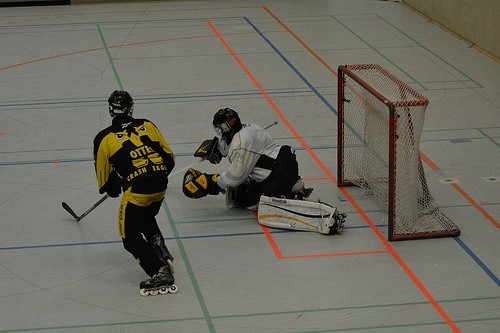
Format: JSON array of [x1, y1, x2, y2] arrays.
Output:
[[107, 90, 135, 117], [212, 107, 243, 139]]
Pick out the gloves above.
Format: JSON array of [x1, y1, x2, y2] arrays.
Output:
[[193, 135, 228, 165], [181, 166, 226, 200], [104, 170, 122, 198]]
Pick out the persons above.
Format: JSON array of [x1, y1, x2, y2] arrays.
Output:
[[93, 90, 178, 296], [182, 108, 314, 214]]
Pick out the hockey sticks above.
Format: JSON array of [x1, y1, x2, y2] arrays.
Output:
[[168, 121, 277, 179], [61, 187, 123, 222]]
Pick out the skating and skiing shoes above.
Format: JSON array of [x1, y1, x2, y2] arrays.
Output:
[[156, 244, 176, 274], [292, 176, 314, 200], [326, 206, 348, 235], [138, 262, 178, 296]]
